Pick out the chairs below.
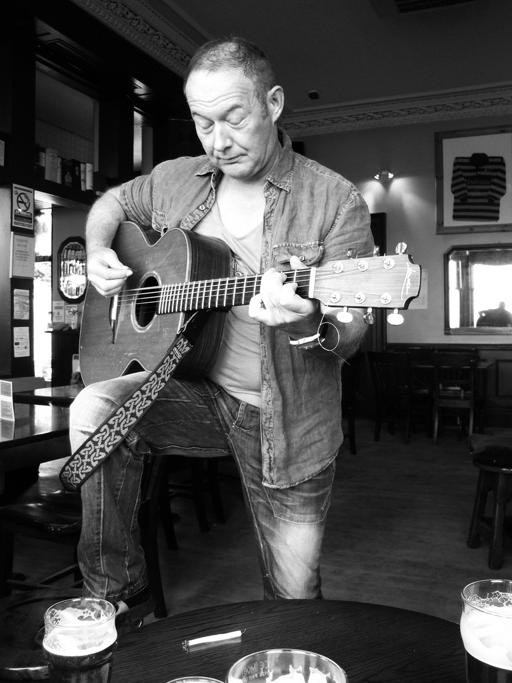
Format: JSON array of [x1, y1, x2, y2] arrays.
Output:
[[341, 351, 381, 454], [366, 346, 482, 442]]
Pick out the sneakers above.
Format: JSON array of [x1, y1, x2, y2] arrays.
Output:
[[35, 586, 157, 644]]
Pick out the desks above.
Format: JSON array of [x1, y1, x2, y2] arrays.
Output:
[[373, 358, 497, 433], [13, 598, 509, 680]]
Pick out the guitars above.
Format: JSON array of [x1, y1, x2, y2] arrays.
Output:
[[79, 221, 421, 388]]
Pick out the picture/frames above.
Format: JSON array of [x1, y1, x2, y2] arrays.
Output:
[[432, 122, 512, 235], [57, 235, 92, 305]]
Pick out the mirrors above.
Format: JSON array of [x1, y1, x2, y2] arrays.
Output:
[[442, 242, 512, 336]]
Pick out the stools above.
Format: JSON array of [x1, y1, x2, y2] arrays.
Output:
[[466, 447, 512, 569]]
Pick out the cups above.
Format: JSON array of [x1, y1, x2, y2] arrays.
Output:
[[40, 596, 119, 682], [455, 576, 512, 683], [226, 646, 347, 682]]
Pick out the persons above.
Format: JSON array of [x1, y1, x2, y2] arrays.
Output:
[[70, 34, 383, 633]]
